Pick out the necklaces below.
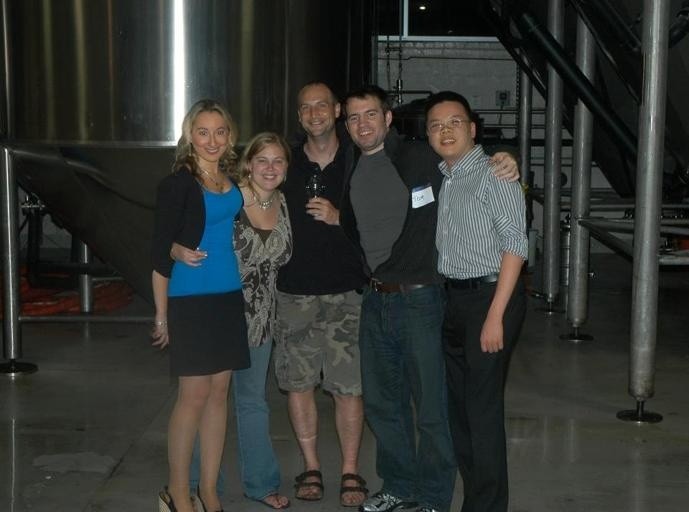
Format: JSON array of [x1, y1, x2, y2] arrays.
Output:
[[248, 172, 280, 211], [199, 166, 227, 193]]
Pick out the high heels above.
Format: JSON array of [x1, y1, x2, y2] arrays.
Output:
[[190, 484, 223, 512], [158, 486, 177, 512]]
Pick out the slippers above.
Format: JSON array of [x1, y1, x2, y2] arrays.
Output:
[[243, 493, 290, 509]]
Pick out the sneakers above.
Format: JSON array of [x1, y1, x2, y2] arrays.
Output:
[[358, 489, 419, 512]]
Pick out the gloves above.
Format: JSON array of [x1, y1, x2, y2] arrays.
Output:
[[445, 274, 499, 289]]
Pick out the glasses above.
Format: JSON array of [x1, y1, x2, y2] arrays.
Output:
[[427, 118, 470, 133]]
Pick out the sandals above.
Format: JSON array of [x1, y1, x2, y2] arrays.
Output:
[[294, 470, 324, 501], [340, 473, 369, 507]]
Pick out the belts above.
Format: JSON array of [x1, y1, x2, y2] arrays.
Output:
[[369, 277, 440, 292]]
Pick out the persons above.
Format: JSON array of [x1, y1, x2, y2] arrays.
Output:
[[338, 83, 522, 511], [275, 79, 370, 508], [151, 99, 252, 511], [422, 89, 529, 511], [169, 131, 293, 509]]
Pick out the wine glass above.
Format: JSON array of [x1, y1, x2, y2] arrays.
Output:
[[305, 176, 326, 216]]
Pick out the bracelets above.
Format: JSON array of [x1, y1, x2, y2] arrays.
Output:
[[154, 320, 168, 328], [170, 244, 176, 262]]
